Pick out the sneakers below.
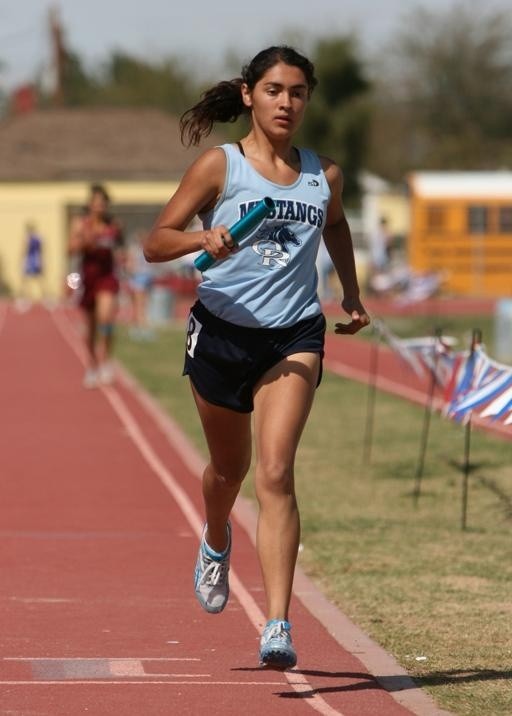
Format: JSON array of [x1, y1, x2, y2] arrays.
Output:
[[192, 516, 232, 615], [81, 359, 116, 390], [257, 618, 298, 669]]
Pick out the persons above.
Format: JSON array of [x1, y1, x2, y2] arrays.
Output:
[[14, 219, 54, 309], [141, 43, 373, 670], [67, 184, 130, 390], [126, 214, 399, 329]]
